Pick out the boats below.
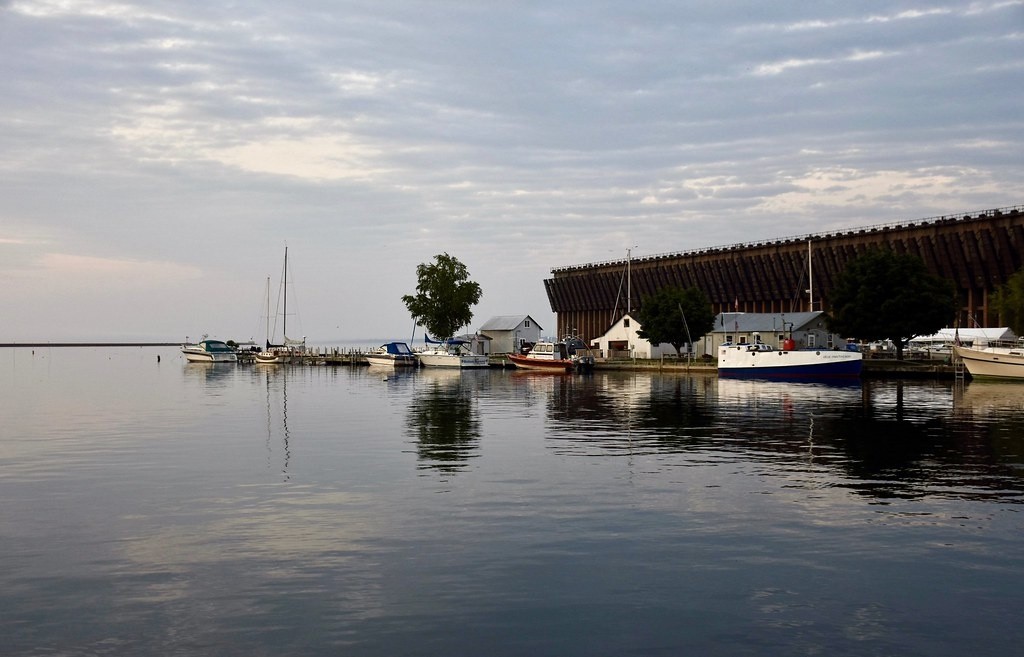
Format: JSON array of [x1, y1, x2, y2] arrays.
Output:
[[506, 338, 597, 375], [254, 351, 279, 363], [954, 345, 1024, 381], [412, 341, 491, 367], [180, 339, 239, 362], [364, 341, 424, 367], [716, 341, 864, 384]]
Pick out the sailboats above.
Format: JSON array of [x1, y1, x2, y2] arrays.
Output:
[[249, 245, 308, 354]]
